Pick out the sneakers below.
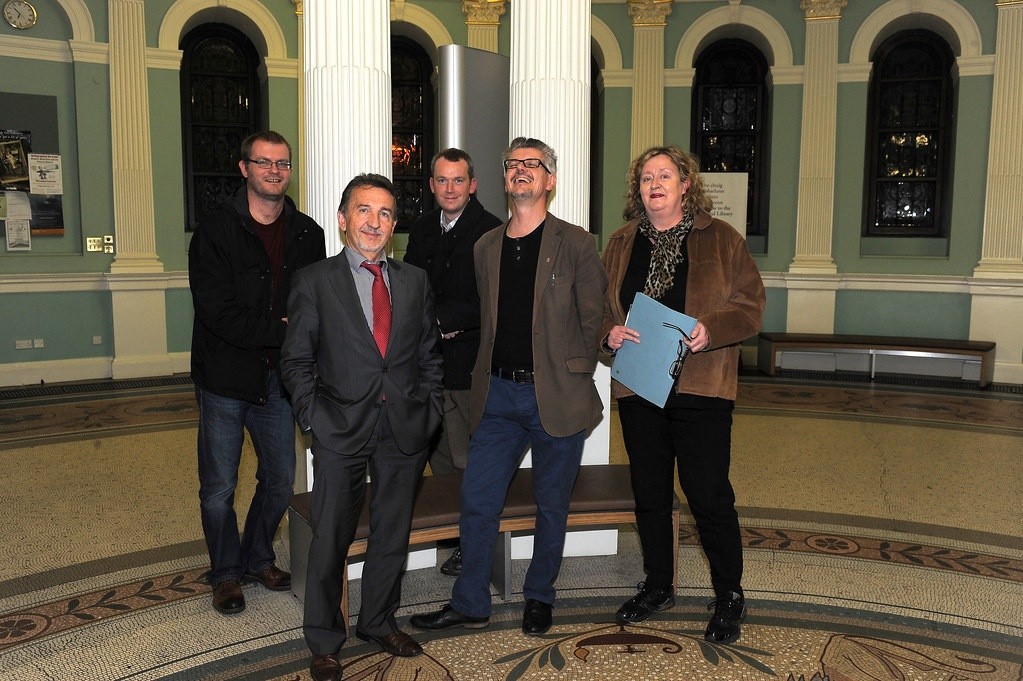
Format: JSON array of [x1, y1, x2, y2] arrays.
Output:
[[441, 548, 462, 576], [617, 580, 675, 623], [705, 590, 747, 644]]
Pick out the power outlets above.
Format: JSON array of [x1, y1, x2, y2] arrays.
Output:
[[15, 340, 32, 348], [34, 339, 43, 348]]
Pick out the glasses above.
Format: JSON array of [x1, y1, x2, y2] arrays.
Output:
[[504, 158, 551, 174], [243, 159, 292, 171], [663, 321, 693, 379]]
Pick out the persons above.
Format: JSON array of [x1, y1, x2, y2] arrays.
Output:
[[280, 172, 443, 681], [407, 137, 608, 635], [403, 148, 505, 574], [596, 145, 766, 643], [188, 129, 326, 616]]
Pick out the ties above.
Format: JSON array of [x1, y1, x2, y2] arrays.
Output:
[[361, 260, 392, 401]]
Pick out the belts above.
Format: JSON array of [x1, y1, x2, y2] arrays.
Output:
[[491, 367, 539, 382]]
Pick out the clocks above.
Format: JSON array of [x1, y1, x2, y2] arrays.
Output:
[[2, 0, 37, 29]]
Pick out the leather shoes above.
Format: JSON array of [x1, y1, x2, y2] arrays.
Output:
[[244, 565, 292, 590], [358, 628, 423, 656], [213, 580, 245, 614], [310, 651, 343, 681], [408, 603, 489, 631], [522, 599, 551, 636]]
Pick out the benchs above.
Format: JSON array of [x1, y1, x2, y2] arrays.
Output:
[[290, 464, 680, 638], [757, 331, 997, 386]]
